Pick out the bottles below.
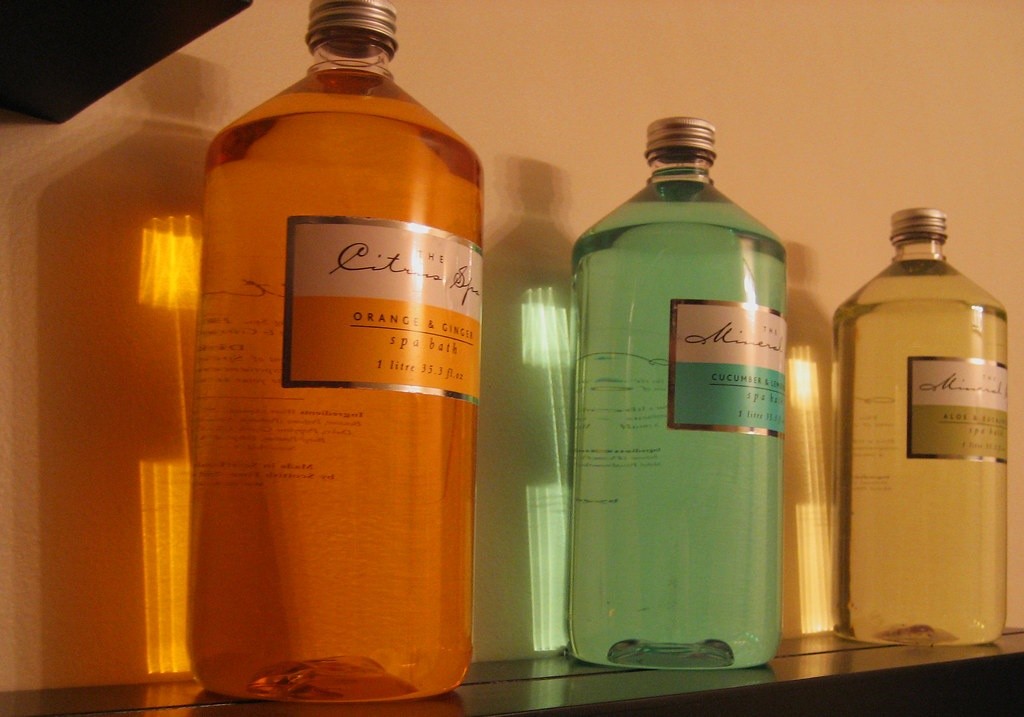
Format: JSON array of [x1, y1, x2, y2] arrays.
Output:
[[192, 0, 487, 702], [833, 209, 1012, 650], [565, 118, 786, 670]]
